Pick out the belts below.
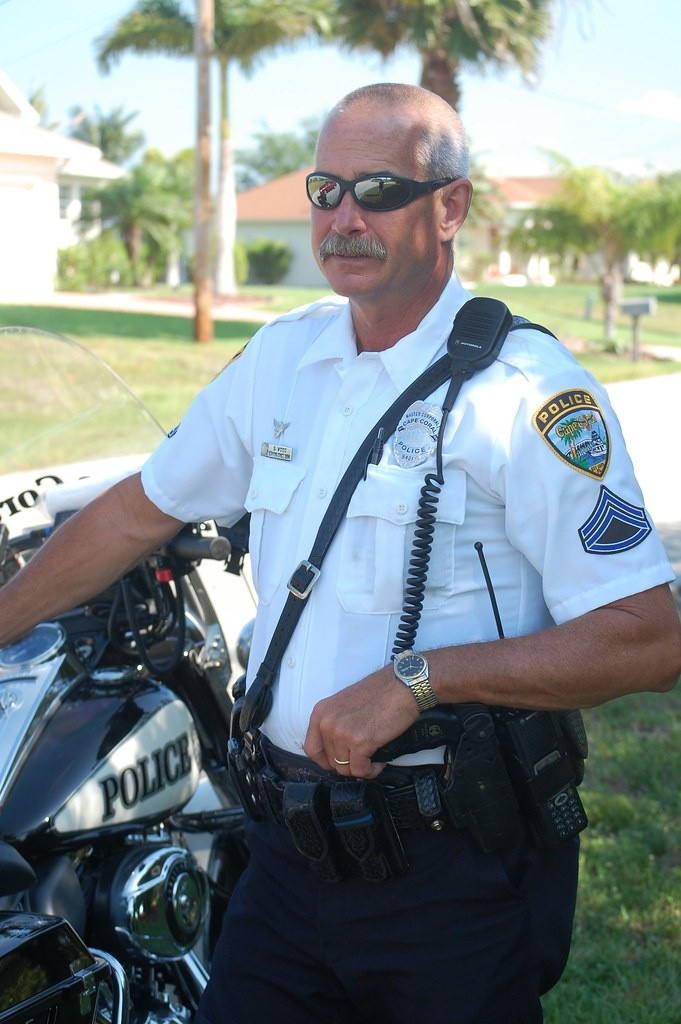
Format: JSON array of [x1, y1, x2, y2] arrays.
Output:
[[255, 744, 368, 791]]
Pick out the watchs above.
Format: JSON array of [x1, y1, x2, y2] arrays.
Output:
[[393, 649, 437, 711]]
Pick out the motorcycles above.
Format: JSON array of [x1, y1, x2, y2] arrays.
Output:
[[0, 326, 255, 1024]]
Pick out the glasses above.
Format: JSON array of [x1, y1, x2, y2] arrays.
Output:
[[306, 171, 461, 211]]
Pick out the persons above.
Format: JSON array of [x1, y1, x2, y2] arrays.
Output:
[[0, 82, 681, 1024]]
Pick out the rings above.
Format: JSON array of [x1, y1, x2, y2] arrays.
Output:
[[334, 756, 350, 765]]
